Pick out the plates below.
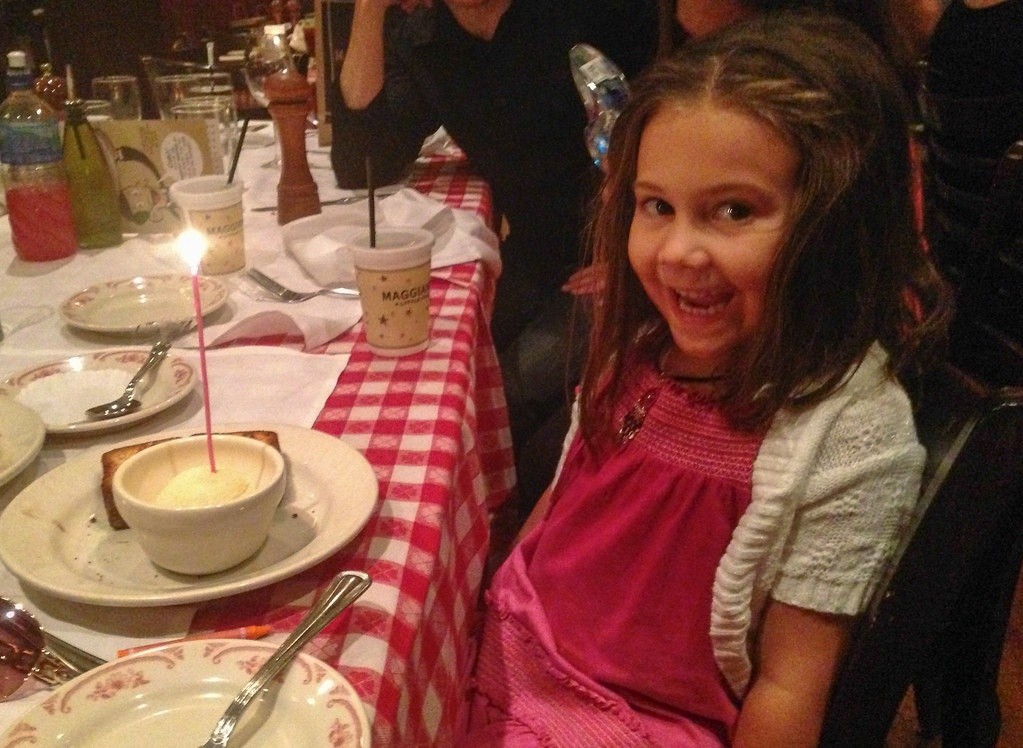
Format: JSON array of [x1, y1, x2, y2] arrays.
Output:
[[0, 639, 372, 748], [0, 382, 46, 487], [60, 274, 228, 333], [2, 346, 197, 433], [0, 422, 378, 605]]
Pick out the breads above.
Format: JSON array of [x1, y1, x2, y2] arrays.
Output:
[[100, 429, 280, 529]]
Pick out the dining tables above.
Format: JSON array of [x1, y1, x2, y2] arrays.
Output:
[[1, 120, 518, 748]]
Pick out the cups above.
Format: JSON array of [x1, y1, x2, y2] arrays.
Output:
[[91, 75, 140, 123], [142, 57, 194, 119], [157, 73, 235, 162], [79, 100, 111, 121], [349, 228, 436, 356], [170, 175, 244, 275], [112, 434, 285, 574]]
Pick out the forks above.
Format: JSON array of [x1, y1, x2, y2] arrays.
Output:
[[243, 269, 360, 301], [259, 149, 331, 170]]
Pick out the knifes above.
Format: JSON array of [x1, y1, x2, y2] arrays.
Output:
[[251, 194, 392, 210]]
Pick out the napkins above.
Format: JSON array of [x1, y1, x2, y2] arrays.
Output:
[[280, 186, 502, 289]]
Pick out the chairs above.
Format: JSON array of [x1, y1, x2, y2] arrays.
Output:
[[817, 340, 1022, 748]]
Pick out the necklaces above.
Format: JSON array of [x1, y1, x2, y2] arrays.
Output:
[[617, 339, 732, 446]]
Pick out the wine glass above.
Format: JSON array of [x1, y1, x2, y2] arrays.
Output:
[[246, 35, 290, 169]]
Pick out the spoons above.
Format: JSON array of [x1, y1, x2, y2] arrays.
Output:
[[86, 340, 172, 418]]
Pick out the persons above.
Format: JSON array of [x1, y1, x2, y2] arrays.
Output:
[[454, 14, 947, 748], [328, 0, 1023, 747]]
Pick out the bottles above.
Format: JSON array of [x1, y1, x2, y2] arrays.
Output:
[[62, 100, 124, 248], [0, 51, 76, 260], [264, 24, 294, 71]]
